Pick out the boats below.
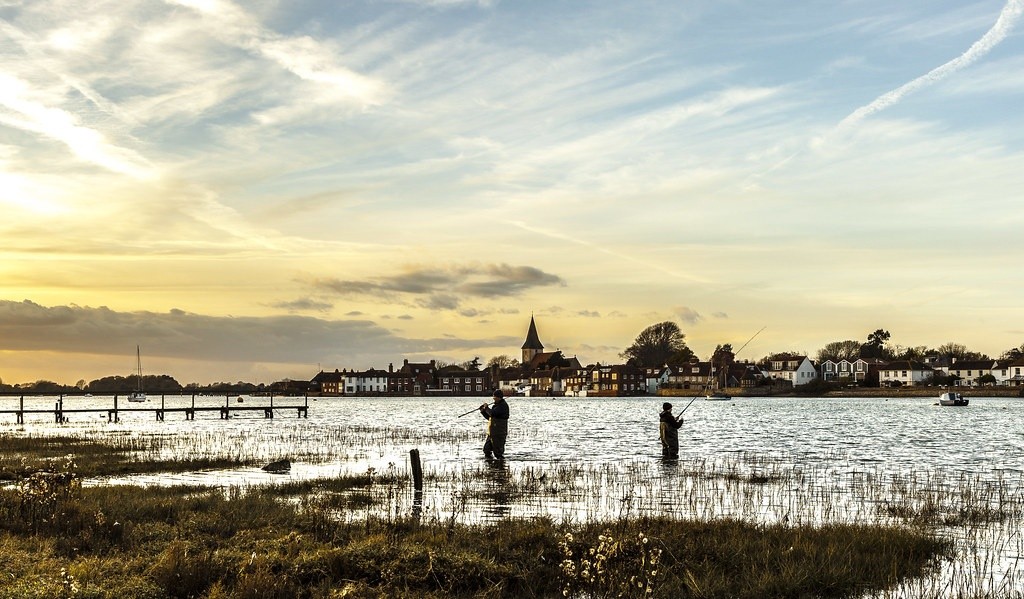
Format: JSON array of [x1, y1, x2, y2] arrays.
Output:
[[939, 392, 970, 406], [706, 392, 732, 400]]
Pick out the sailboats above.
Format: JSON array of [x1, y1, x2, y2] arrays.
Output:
[[127, 344, 147, 402]]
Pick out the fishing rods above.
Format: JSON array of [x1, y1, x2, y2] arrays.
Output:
[[656, 324, 768, 442], [457, 391, 518, 419]]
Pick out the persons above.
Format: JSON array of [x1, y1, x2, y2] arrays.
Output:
[[659, 402, 683, 459], [480, 390, 509, 459]]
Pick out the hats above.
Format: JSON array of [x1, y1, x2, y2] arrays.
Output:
[[493, 390, 503, 398], [663, 403, 672, 411]]
